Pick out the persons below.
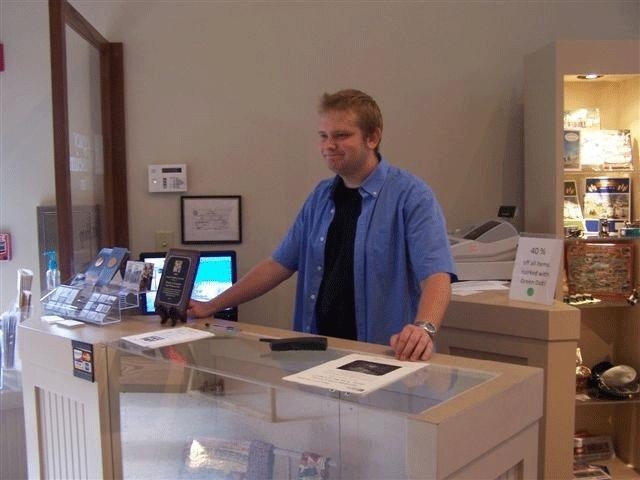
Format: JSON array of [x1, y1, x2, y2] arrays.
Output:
[[187, 89, 459, 362]]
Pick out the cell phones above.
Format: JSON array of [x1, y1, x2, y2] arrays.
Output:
[[259, 336, 328, 351]]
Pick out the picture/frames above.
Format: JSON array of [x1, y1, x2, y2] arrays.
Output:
[[181, 195, 242, 245]]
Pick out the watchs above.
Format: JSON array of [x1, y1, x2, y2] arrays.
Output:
[[415, 320, 438, 339]]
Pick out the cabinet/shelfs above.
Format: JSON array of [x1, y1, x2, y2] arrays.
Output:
[[105, 318, 545, 480], [522, 35, 640, 480]]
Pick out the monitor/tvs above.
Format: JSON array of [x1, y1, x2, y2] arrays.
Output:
[[461, 219, 502, 241], [139, 250, 238, 323]]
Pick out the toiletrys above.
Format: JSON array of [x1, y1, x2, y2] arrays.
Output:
[[43, 250, 60, 298]]
